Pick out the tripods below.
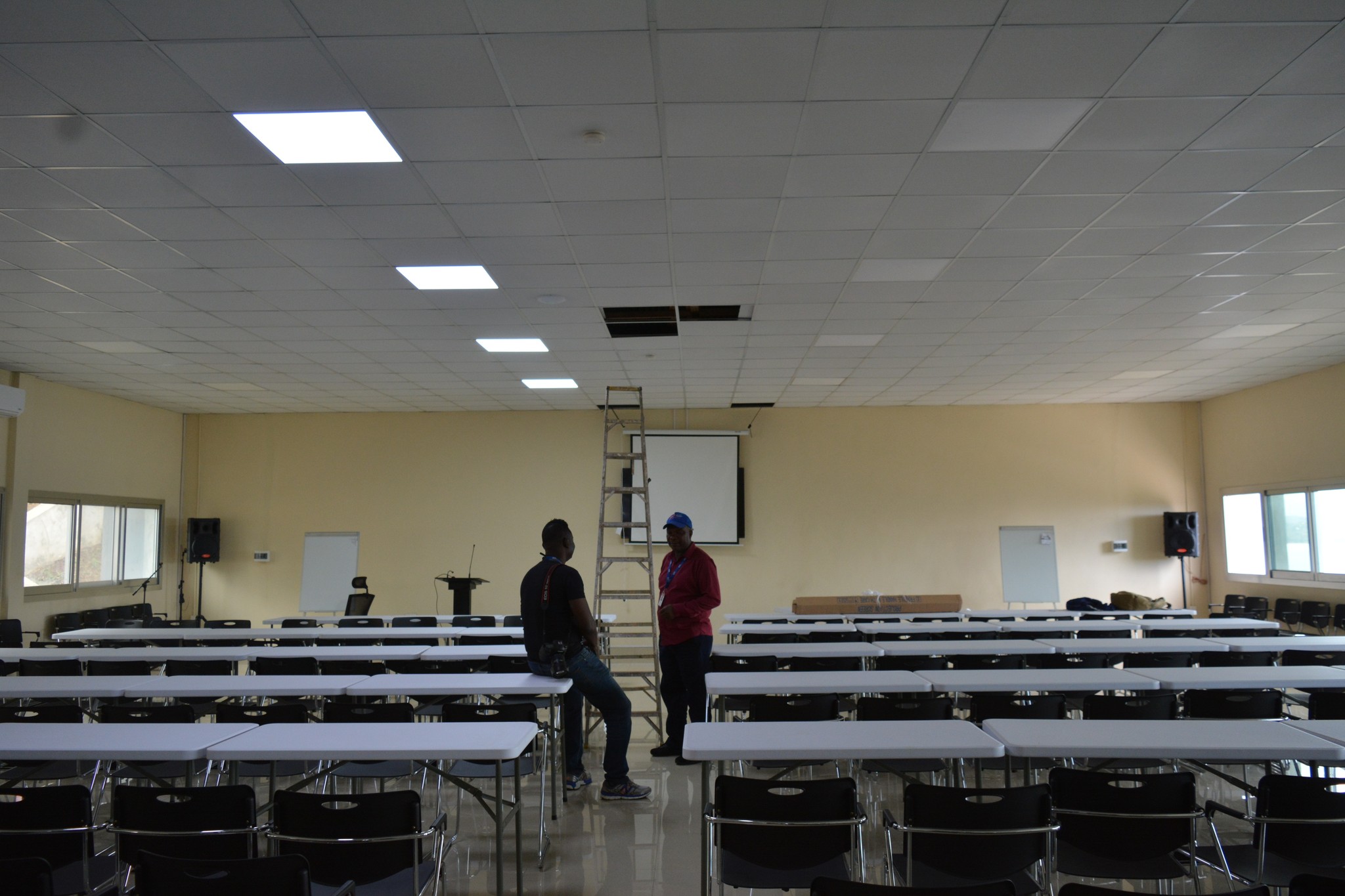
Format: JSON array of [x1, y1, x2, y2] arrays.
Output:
[[195, 562, 209, 646]]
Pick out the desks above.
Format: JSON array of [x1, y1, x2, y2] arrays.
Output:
[[0, 603, 1345, 896]]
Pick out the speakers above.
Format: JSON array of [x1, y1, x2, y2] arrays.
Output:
[[187, 518, 220, 565], [1164, 511, 1199, 559]]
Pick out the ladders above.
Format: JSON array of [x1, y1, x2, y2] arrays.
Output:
[[583, 386, 663, 754]]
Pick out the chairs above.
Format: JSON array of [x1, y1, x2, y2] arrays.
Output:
[[106, 785, 275, 896], [265, 790, 447, 895], [0, 784, 126, 896], [703, 774, 867, 896], [883, 784, 1061, 895], [135, 849, 356, 895], [0, 576, 1345, 867], [1048, 767, 1205, 896], [1170, 774, 1345, 896]]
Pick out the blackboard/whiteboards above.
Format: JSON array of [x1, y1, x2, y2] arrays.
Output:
[[998, 525, 1060, 603], [298, 532, 360, 612]]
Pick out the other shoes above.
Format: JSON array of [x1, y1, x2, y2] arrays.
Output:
[[650, 742, 681, 756], [674, 756, 702, 764]]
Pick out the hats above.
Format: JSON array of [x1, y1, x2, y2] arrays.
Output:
[[662, 512, 693, 530]]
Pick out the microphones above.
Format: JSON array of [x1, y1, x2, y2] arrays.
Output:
[[469, 545, 475, 578], [446, 570, 454, 578]]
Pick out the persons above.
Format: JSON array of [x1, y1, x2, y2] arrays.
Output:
[[650, 512, 722, 766], [520, 519, 652, 800]]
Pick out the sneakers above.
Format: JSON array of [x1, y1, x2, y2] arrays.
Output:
[[565, 770, 592, 789], [600, 776, 652, 800]]
